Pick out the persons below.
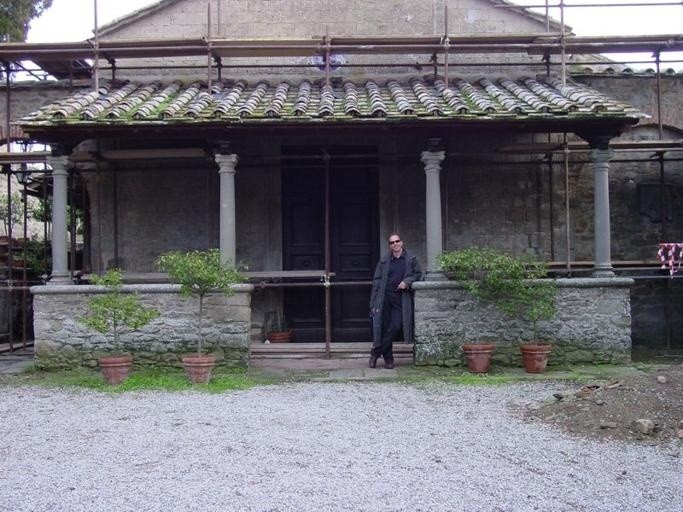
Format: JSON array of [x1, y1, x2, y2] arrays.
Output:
[[369, 234, 422, 369]]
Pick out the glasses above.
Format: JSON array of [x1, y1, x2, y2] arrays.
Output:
[[389, 239, 400, 245]]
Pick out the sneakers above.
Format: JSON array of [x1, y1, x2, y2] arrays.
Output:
[[383, 355, 394, 370], [369, 349, 382, 369]]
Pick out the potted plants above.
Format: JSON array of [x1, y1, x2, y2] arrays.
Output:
[[0, 186, 33, 267], [73, 249, 251, 384], [435, 245, 559, 374]]
